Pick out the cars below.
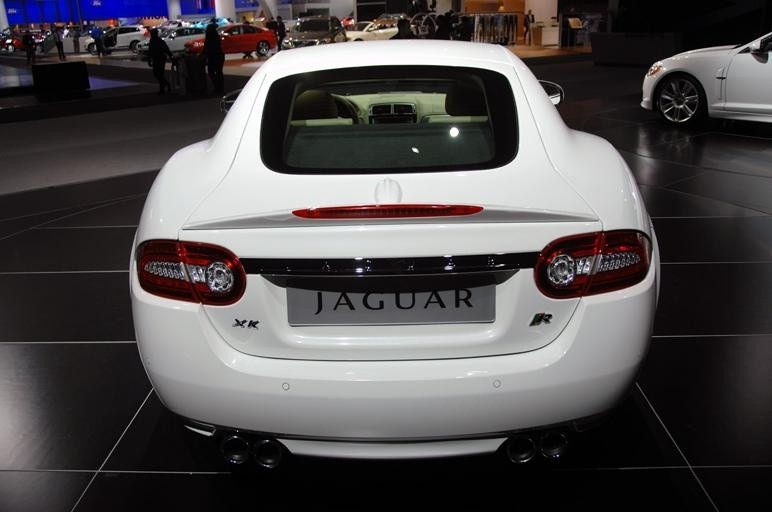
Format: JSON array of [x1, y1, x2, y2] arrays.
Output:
[[126, 34, 668, 472], [281, 15, 346, 51], [344, 19, 422, 41], [638, 27, 772, 131], [183, 23, 279, 57], [0, 8, 230, 56]]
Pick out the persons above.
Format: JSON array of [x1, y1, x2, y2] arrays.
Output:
[[39, 24, 45, 33], [27, 37, 36, 63], [522, 9, 535, 40], [22, 30, 31, 58], [242, 15, 251, 24], [458, 16, 472, 41], [268, 17, 278, 35], [53, 26, 66, 60], [198, 23, 226, 96], [434, 14, 451, 39], [90, 25, 105, 56], [176, 22, 185, 36], [388, 18, 419, 39], [145, 27, 175, 94], [73, 28, 81, 53], [341, 12, 356, 27], [275, 15, 286, 51]]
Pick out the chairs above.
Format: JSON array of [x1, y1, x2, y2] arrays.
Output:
[[292, 89, 358, 126], [421, 77, 489, 124]]
